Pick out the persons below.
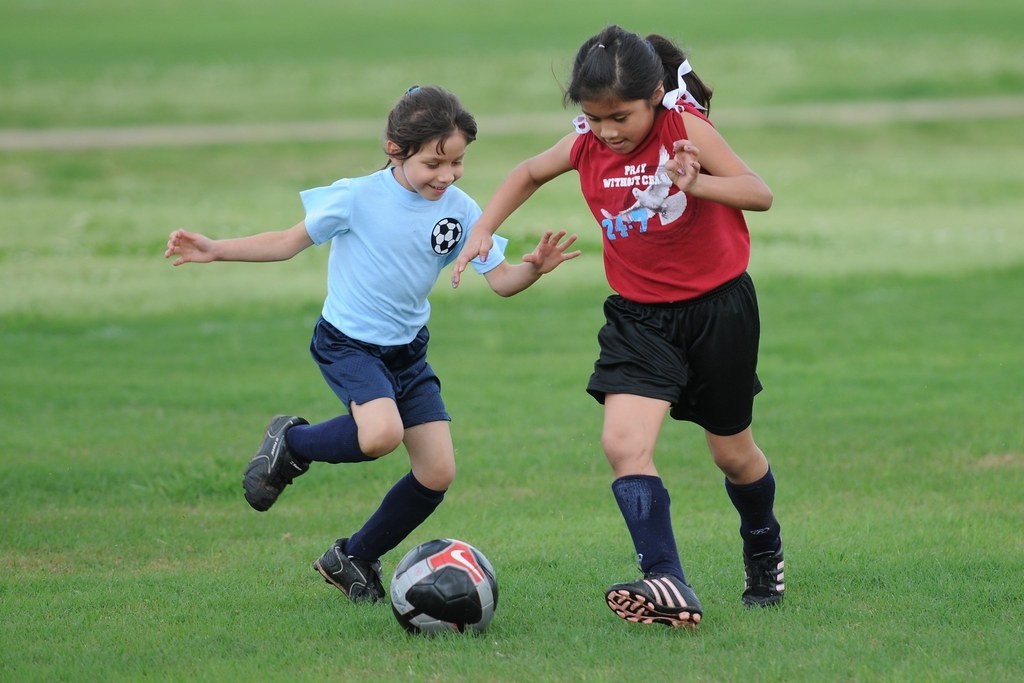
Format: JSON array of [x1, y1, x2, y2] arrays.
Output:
[[452, 25, 785, 627], [165, 83, 582, 606]]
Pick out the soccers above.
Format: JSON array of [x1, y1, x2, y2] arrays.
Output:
[[390, 538, 499, 636]]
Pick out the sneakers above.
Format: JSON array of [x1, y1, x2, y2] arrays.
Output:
[[604, 573, 703, 635], [739, 533, 787, 608], [241, 412, 312, 511], [310, 538, 386, 606]]
